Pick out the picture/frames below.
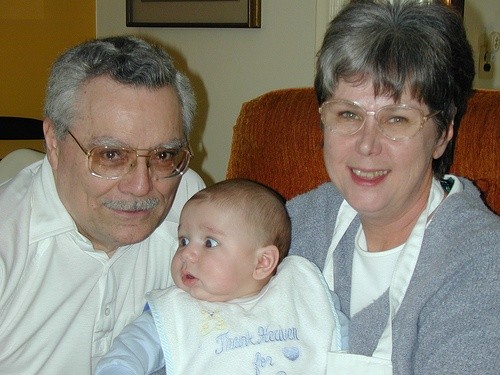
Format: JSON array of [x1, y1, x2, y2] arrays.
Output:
[[125, 0, 262, 29]]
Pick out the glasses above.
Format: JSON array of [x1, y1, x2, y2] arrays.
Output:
[[318, 99, 443, 142], [67, 130, 194, 181]]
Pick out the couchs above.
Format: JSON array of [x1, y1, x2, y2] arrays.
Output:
[[222, 86, 500, 216]]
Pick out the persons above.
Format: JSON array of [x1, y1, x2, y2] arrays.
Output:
[[285, 0, 500, 375], [92, 177, 349, 375], [0, 34, 206, 374]]
